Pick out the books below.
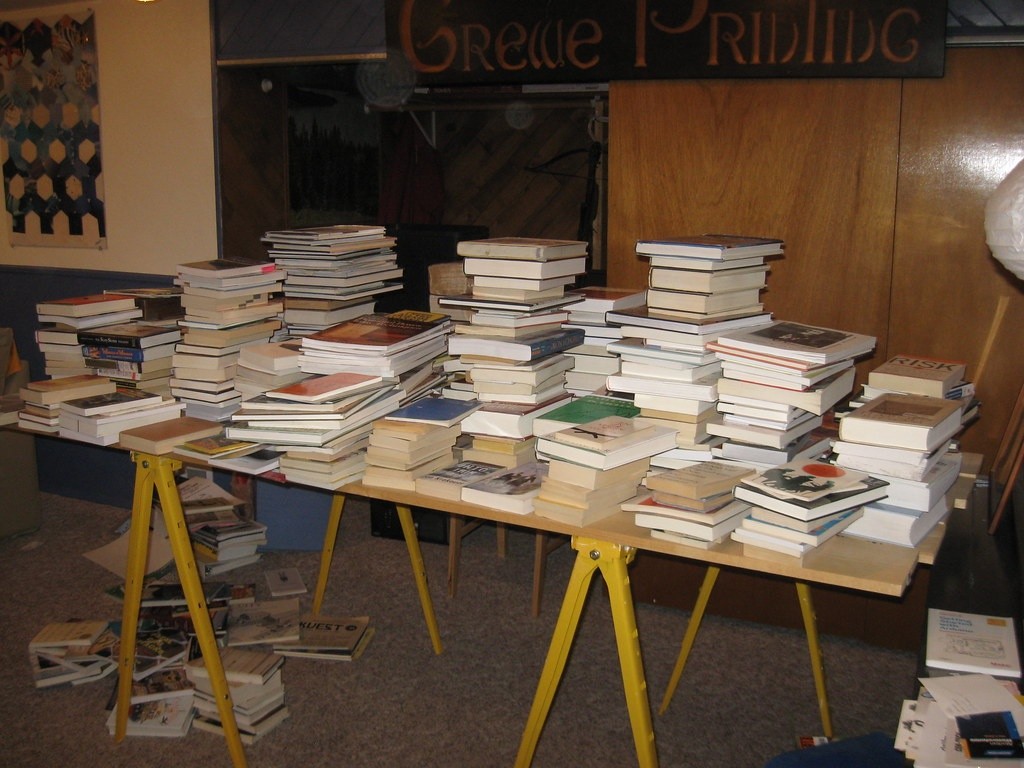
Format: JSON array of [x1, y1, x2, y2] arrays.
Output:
[[0, 224, 978, 556], [28, 478, 375, 745], [893, 608, 1023, 767]]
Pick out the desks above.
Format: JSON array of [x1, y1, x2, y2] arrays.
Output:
[[0, 422, 986, 768]]
